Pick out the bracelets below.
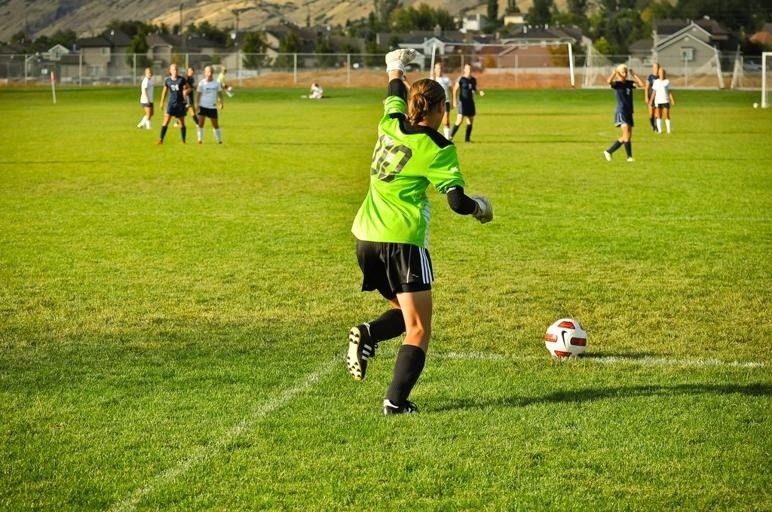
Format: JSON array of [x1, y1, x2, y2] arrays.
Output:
[[473, 205, 478, 216]]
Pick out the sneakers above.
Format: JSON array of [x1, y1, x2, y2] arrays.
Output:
[[346, 322, 378, 381], [604, 150, 613, 162], [383, 398, 420, 417]]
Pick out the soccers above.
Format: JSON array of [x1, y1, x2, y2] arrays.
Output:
[[544, 318, 587, 358]]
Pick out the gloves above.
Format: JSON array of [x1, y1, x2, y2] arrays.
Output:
[[385, 48, 416, 75], [473, 195, 494, 224]]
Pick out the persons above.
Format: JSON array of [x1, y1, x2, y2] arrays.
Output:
[[643, 63, 664, 133], [344, 47, 493, 419], [433, 62, 455, 142], [309, 81, 324, 99], [649, 68, 677, 134], [450, 62, 486, 144], [136, 62, 238, 146], [602, 65, 645, 162]]
